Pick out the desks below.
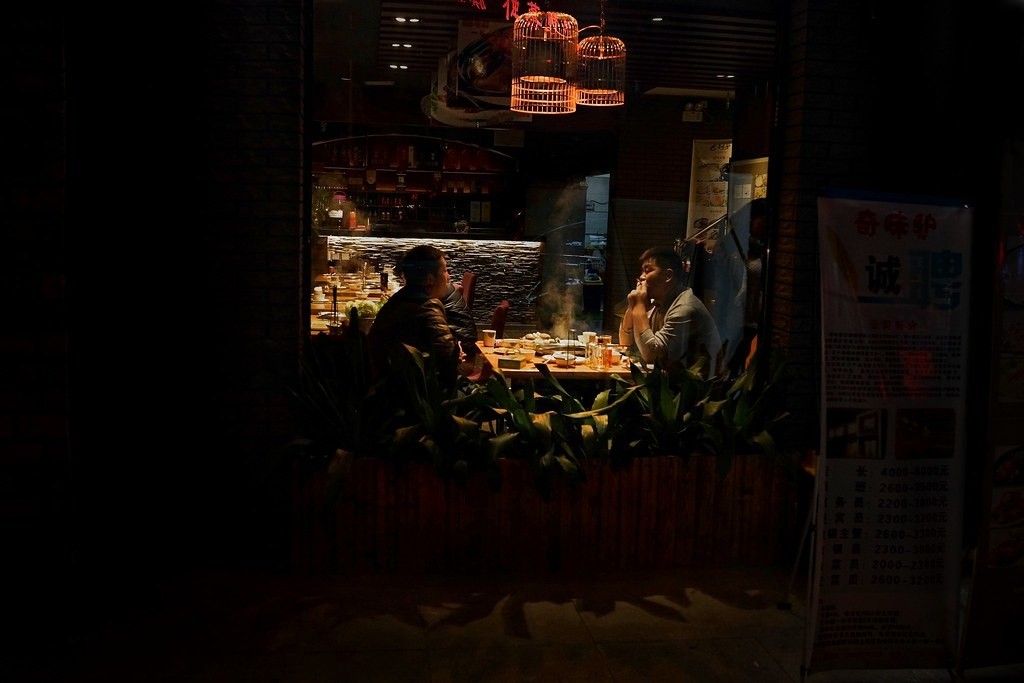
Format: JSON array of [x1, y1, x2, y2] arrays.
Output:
[[475, 336, 632, 409]]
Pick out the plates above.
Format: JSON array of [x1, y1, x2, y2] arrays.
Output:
[[313, 299, 329, 302], [543, 354, 556, 364], [575, 356, 585, 364], [494, 347, 515, 354]]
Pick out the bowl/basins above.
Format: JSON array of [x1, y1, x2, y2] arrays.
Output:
[[612, 354, 622, 365], [555, 354, 576, 365], [577, 335, 599, 343], [314, 294, 325, 300], [355, 292, 369, 299], [517, 349, 536, 362]]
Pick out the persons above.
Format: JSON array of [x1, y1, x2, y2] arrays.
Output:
[[364, 245, 475, 397], [619, 246, 724, 382]]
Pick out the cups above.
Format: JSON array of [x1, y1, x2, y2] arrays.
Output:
[[482, 329, 496, 347], [600, 335, 612, 344], [601, 349, 612, 368], [583, 331, 596, 337]]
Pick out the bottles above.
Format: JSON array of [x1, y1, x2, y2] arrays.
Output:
[[585, 335, 598, 366], [325, 194, 351, 228], [354, 193, 405, 223], [592, 338, 609, 371]]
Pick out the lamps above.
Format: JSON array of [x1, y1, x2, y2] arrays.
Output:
[[681, 104, 703, 122]]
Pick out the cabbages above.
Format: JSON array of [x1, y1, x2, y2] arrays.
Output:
[[347, 300, 387, 317]]
